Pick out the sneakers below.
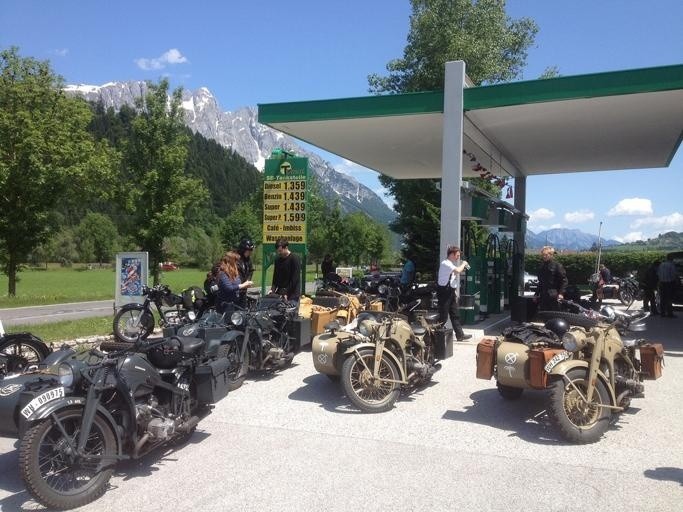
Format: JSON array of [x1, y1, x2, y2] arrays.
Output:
[[644, 305, 678, 318], [457, 334, 472, 341]]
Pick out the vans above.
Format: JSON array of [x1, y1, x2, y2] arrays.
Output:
[[657, 252, 683, 305]]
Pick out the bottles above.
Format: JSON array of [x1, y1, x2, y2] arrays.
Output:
[[461, 259, 472, 271]]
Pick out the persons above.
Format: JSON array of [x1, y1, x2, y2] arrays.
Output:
[[532, 244, 568, 311], [400, 247, 418, 286], [321, 254, 342, 284], [435, 246, 472, 341], [599, 265, 611, 282], [642, 260, 661, 315], [272, 237, 301, 309], [204, 237, 255, 308], [658, 254, 679, 318], [211, 251, 254, 312]]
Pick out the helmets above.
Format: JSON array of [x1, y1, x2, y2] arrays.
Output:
[[357, 313, 377, 329], [239, 240, 254, 254], [546, 318, 569, 336]]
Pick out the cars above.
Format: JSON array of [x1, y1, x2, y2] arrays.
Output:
[[523, 271, 539, 291]]
